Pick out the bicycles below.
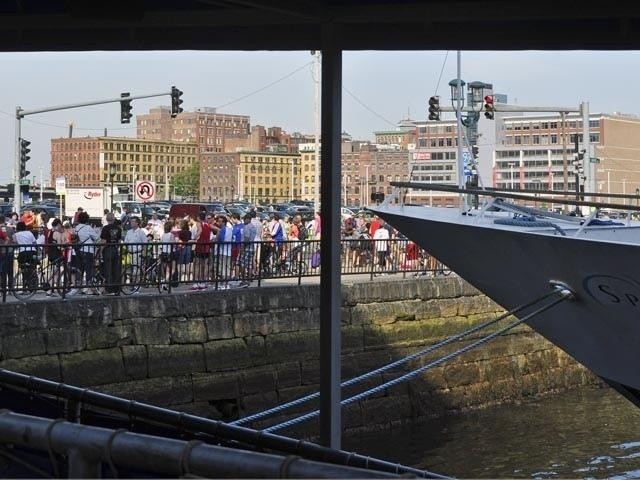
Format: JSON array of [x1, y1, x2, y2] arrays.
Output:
[[441, 262, 452, 276]]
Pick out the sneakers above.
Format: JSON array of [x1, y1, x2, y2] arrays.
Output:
[[46, 289, 120, 297], [213, 284, 231, 290], [236, 281, 250, 288], [189, 283, 206, 290], [130, 286, 141, 291]]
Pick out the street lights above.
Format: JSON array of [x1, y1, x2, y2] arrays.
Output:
[[447, 78, 484, 210], [605, 168, 612, 218], [290, 159, 294, 200], [108, 161, 116, 213], [164, 161, 171, 202], [428, 173, 433, 207], [508, 163, 516, 189], [343, 174, 349, 206], [130, 163, 137, 201], [236, 164, 242, 194], [364, 165, 371, 207]]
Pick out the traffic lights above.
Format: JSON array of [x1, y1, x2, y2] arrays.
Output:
[[483, 94, 495, 120], [429, 95, 439, 120], [119, 92, 133, 123], [21, 139, 32, 177], [571, 152, 579, 176], [171, 85, 184, 119]]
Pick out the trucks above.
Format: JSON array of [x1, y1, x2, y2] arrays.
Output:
[[63, 186, 143, 223]]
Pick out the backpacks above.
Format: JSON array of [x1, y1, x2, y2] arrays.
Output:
[[71, 225, 89, 249], [298, 221, 308, 240]]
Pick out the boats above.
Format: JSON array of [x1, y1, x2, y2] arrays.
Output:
[[366, 179, 640, 408]]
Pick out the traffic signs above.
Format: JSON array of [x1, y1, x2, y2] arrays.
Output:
[[591, 158, 601, 163], [20, 179, 31, 185]]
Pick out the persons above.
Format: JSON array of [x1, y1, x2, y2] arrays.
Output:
[[1, 207, 440, 296]]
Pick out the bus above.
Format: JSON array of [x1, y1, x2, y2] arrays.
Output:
[[531, 205, 640, 222]]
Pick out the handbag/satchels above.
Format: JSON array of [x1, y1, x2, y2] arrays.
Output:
[[47, 231, 58, 262]]
